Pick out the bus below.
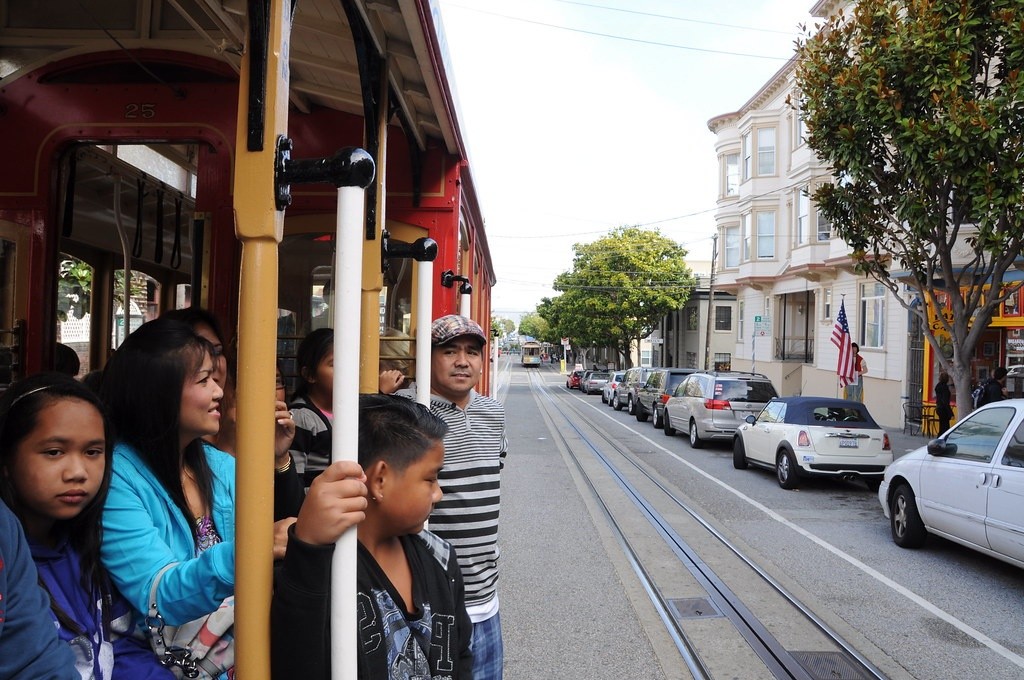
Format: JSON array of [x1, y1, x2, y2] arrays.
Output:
[[521, 340, 543, 368]]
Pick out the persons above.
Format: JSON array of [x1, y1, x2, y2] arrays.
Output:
[[934, 372, 955, 438], [979, 366, 1009, 406], [286, 328, 406, 435], [0, 306, 307, 679], [386, 314, 508, 680], [269, 392, 477, 680]]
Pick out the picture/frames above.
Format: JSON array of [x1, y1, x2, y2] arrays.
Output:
[[976, 366, 989, 382], [983, 341, 995, 357], [941, 344, 954, 361], [969, 346, 978, 362]]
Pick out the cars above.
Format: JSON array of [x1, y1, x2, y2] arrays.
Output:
[[662, 369, 781, 449], [732, 396, 895, 494], [876, 396, 1024, 570], [602, 370, 627, 407], [566, 368, 611, 395], [635, 367, 720, 429], [613, 367, 658, 415]]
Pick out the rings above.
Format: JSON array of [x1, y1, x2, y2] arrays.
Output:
[[289, 411, 294, 419]]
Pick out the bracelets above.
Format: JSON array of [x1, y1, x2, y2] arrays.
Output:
[[276, 455, 291, 473]]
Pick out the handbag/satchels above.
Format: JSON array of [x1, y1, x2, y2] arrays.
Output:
[[936, 405, 954, 420], [145, 562, 234, 680]]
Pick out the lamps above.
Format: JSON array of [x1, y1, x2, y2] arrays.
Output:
[[798, 304, 803, 315]]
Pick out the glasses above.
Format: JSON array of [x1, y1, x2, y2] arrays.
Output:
[[276, 377, 286, 390]]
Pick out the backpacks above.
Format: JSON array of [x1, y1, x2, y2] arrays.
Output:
[[972, 381, 1002, 409]]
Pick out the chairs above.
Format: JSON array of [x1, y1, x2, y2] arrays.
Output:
[[927, 404, 956, 438], [903, 401, 923, 435]]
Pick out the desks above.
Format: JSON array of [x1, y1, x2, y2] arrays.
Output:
[[908, 404, 937, 437]]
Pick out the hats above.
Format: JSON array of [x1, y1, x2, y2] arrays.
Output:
[[432, 315, 486, 346]]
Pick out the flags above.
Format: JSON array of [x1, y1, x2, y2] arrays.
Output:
[[830, 299, 855, 388]]
